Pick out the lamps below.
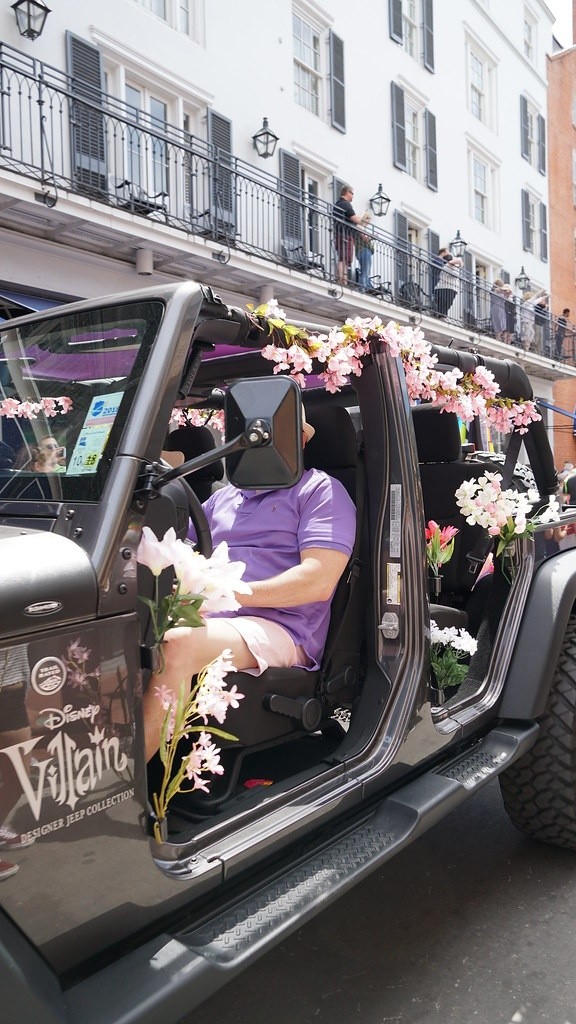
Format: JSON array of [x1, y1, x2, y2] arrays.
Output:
[[369, 183, 391, 216], [450, 230, 468, 257], [10, 0, 52, 41], [136, 248, 153, 276], [259, 285, 274, 306], [514, 266, 530, 289], [251, 117, 279, 158]]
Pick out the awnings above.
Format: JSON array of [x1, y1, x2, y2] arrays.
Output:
[[538, 399, 576, 429]]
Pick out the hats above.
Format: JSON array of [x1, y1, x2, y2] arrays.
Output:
[[300, 402, 316, 442]]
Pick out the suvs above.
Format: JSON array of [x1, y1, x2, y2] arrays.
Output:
[[1, 279, 575, 1024]]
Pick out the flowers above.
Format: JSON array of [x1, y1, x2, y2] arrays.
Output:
[[0, 298, 560, 835]]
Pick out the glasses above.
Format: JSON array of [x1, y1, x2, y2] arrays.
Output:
[[40, 444, 59, 451]]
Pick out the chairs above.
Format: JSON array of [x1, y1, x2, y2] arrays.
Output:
[[196, 408, 365, 742], [280, 239, 325, 278], [400, 282, 432, 311], [466, 311, 492, 333], [187, 206, 236, 245], [166, 424, 224, 502], [109, 177, 170, 224], [508, 330, 550, 356], [407, 402, 503, 627], [354, 269, 392, 295]]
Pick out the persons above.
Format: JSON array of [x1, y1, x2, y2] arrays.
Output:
[[556, 309, 570, 363], [520, 289, 551, 351], [561, 461, 576, 504], [490, 278, 515, 344], [143, 405, 358, 764], [432, 248, 462, 320], [333, 184, 361, 285], [16, 434, 66, 472], [355, 210, 374, 291]]
